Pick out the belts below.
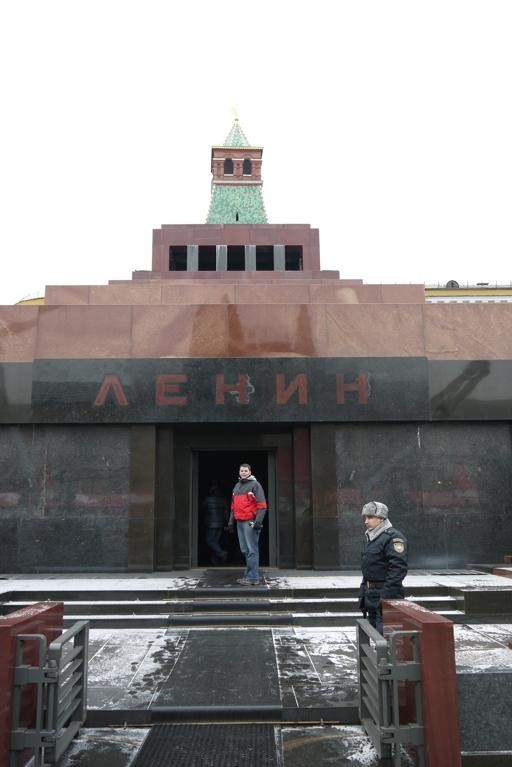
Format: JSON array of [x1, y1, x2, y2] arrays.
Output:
[[366, 581, 385, 589]]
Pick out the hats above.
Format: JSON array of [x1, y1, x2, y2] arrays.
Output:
[[361, 502, 388, 518]]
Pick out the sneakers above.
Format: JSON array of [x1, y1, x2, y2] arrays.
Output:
[[237, 579, 259, 585]]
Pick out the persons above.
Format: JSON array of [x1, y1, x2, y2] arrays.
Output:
[[227, 463, 268, 586], [201, 485, 231, 563], [358, 500, 408, 628]]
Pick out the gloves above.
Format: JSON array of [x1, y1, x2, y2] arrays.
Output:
[[254, 523, 261, 529]]
